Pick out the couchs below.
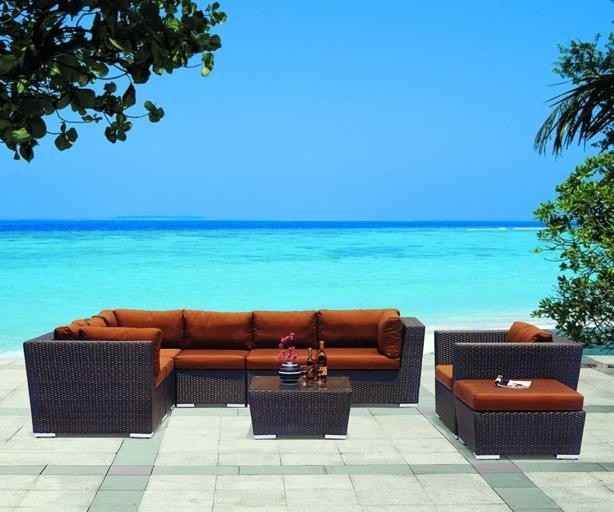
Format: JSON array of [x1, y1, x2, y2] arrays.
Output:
[[23, 307, 426, 462]]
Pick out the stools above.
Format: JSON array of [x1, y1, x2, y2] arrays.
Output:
[[452, 379, 585, 458]]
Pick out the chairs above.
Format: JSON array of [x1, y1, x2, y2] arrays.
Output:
[[433, 321, 585, 459]]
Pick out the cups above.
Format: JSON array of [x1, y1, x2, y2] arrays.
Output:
[[300, 365, 307, 378]]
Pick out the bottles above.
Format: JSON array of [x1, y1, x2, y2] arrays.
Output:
[[305, 347, 315, 379], [315, 340, 329, 388]]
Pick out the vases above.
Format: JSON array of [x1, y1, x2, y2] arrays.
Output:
[[277, 361, 302, 383]]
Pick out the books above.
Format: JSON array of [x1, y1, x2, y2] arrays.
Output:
[[495, 374, 532, 390]]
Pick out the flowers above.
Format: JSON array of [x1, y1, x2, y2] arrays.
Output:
[[272, 331, 299, 363]]
